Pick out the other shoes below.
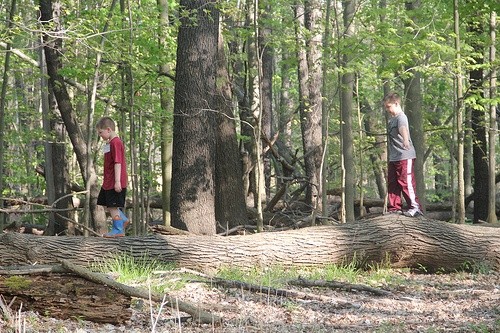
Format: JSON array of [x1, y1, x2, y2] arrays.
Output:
[[403, 209, 420, 217], [382, 209, 403, 215]]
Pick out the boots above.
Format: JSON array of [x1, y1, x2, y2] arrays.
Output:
[[119, 209, 130, 229], [103, 215, 125, 238]]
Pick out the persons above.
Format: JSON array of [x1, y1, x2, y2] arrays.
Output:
[[95, 116, 130, 238], [382, 92, 424, 217]]
